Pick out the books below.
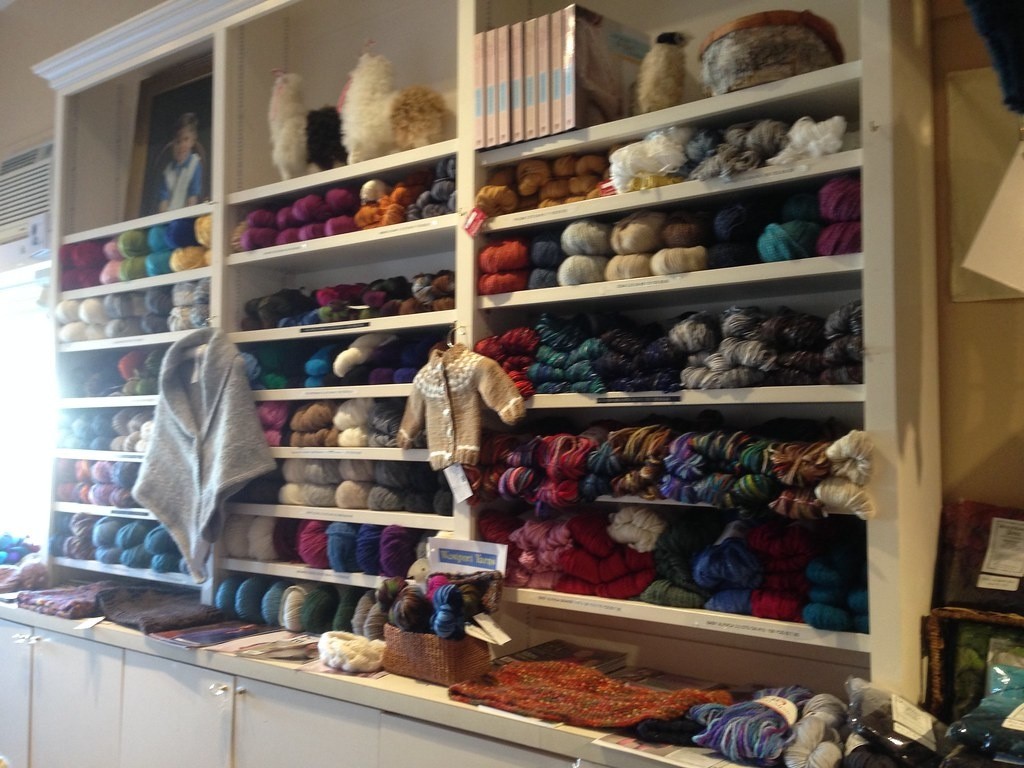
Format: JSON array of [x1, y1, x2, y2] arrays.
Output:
[[474, 3, 652, 153]]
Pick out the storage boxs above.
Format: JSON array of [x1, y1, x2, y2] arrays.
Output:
[[381, 622, 493, 690]]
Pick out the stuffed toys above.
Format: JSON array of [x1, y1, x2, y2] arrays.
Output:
[[337, 51, 456, 165], [635, 31, 707, 114], [269, 69, 349, 181]]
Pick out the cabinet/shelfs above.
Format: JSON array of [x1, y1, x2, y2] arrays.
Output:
[[0, 0, 942, 768]]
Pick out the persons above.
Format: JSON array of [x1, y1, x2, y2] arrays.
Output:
[[156, 112, 202, 214]]
